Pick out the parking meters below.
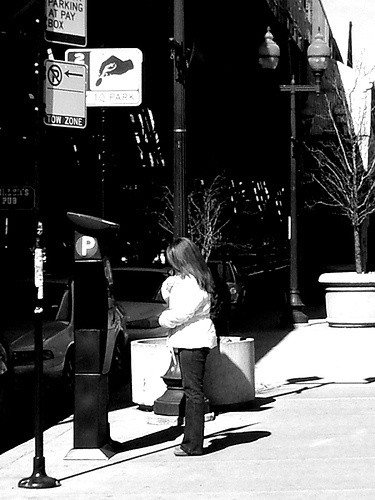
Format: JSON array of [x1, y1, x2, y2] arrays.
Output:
[[65, 211, 119, 451]]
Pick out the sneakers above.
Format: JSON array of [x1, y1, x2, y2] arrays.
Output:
[[174, 449, 188, 455]]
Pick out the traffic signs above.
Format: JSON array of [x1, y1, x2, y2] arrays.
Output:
[[65, 48, 143, 107], [45, 58, 89, 130]]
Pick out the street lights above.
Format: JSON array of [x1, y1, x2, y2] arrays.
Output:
[[251, 18, 338, 325]]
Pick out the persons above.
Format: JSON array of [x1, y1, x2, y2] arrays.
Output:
[[158, 237, 218, 457]]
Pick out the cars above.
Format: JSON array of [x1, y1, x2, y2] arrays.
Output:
[[205, 258, 245, 320], [105, 267, 227, 345], [9, 280, 127, 402]]
